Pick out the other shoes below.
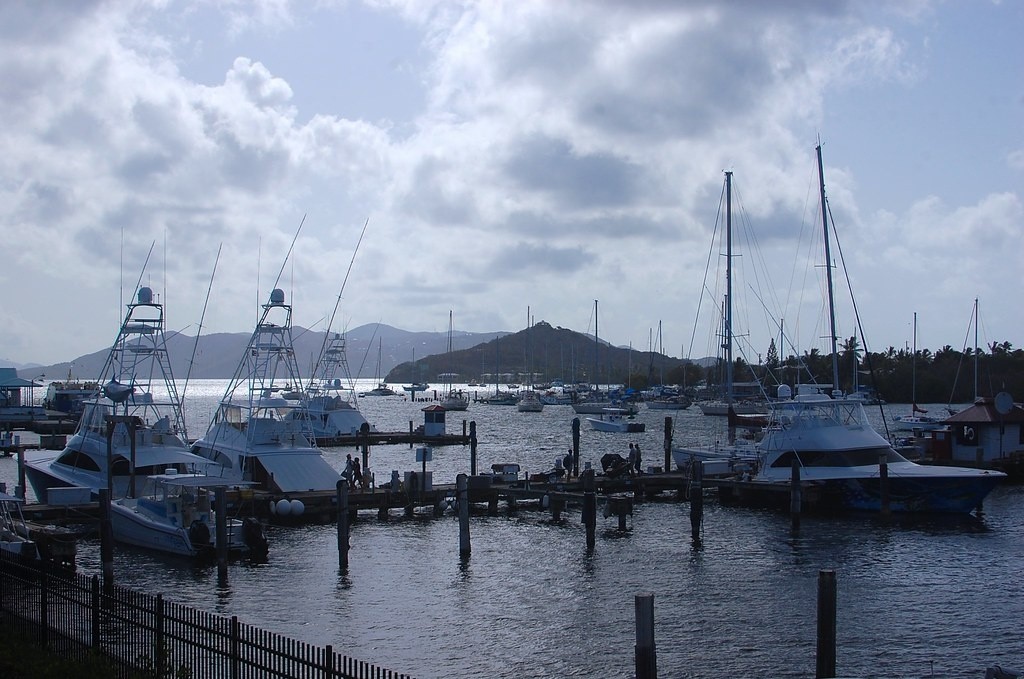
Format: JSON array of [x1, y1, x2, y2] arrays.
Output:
[[641, 470, 644, 475]]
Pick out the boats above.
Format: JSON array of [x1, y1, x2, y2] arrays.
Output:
[[669, 129, 1008, 519], [1, 211, 985, 559]]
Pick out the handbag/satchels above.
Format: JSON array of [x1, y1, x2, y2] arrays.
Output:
[[341, 471, 348, 477]]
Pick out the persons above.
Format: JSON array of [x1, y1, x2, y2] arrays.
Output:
[[566, 450, 573, 482], [346, 454, 363, 491], [628, 443, 644, 477]]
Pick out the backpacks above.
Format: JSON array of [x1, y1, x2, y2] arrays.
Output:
[[563, 455, 570, 469]]
